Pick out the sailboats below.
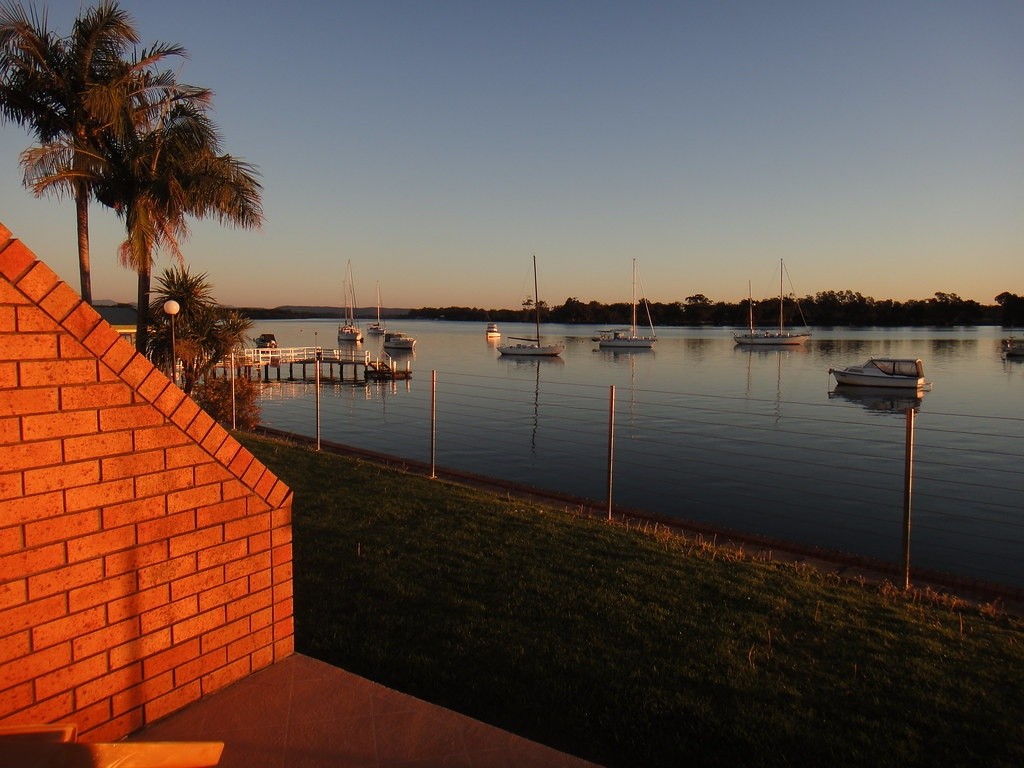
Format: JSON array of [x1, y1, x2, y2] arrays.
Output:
[[732, 257, 813, 345], [338, 257, 362, 342], [599, 256, 659, 347], [495, 255, 566, 357], [367, 280, 387, 333]]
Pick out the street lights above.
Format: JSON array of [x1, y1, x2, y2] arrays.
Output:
[[164, 300, 182, 387]]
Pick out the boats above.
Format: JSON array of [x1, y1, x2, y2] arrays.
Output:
[[485, 322, 501, 337], [383, 332, 416, 348], [830, 356, 925, 389], [592, 327, 633, 342], [363, 361, 411, 380], [256, 334, 278, 350], [1001, 336, 1024, 363]]
